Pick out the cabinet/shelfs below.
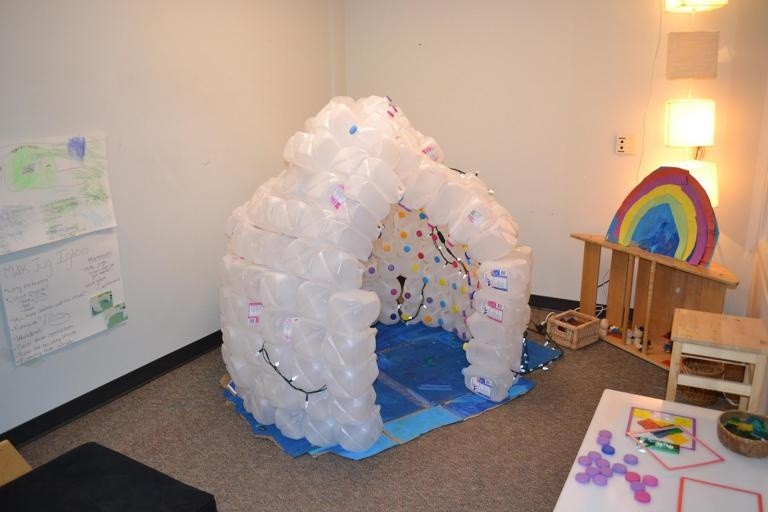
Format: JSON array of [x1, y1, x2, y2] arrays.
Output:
[[569, 233, 738, 371]]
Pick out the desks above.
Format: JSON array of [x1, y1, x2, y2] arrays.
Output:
[[552, 388, 768, 512]]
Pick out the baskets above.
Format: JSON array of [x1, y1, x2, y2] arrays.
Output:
[[546, 309, 600, 351], [680, 357, 744, 407], [718, 410, 767, 460]]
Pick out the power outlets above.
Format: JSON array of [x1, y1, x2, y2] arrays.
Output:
[[616, 135, 633, 156]]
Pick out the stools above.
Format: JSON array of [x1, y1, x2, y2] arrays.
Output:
[[663, 307, 768, 412]]
